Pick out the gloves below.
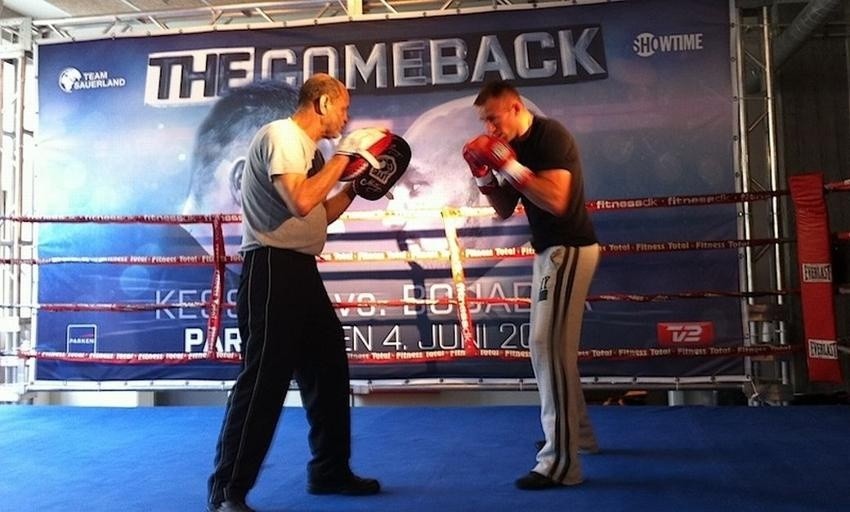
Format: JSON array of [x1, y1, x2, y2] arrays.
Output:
[[470, 134, 536, 191], [460, 139, 501, 196]]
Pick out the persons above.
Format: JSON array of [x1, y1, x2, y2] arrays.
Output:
[[463, 79, 601, 490], [207, 73, 411, 512], [39, 81, 310, 379], [384, 92, 554, 376]]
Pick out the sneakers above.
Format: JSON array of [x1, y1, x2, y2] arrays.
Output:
[[208, 501, 255, 512], [535, 438, 547, 450], [516, 472, 557, 490], [306, 473, 380, 496]]
